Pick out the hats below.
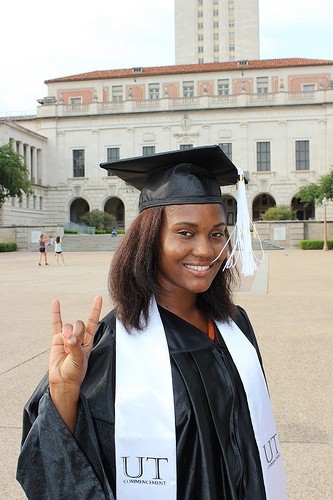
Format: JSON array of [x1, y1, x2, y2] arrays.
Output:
[[97, 143, 267, 275]]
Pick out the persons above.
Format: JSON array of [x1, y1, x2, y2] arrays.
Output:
[[53, 235, 65, 266], [111, 226, 117, 237], [44, 236, 53, 246], [38, 233, 49, 266], [16, 142, 288, 500]]
